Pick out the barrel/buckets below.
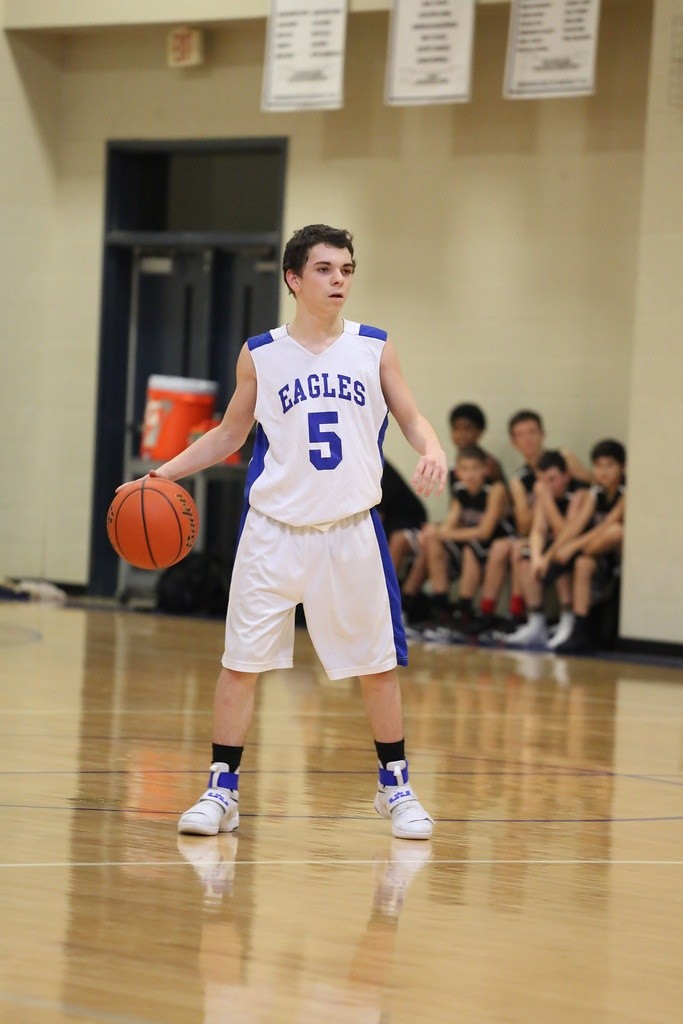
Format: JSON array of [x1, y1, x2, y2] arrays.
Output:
[[135, 375, 217, 466]]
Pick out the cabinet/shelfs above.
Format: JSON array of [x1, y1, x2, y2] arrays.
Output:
[[114, 458, 250, 603]]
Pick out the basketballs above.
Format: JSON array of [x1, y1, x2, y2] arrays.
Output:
[[106, 478, 200, 570]]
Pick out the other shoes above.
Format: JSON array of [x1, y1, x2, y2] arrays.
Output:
[[400, 602, 509, 646]]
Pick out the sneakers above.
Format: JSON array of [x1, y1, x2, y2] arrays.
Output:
[[506, 612, 548, 648], [373, 759, 436, 841], [548, 612, 580, 651], [177, 762, 240, 836]]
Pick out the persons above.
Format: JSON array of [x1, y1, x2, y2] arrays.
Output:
[[417, 445, 517, 640], [503, 448, 591, 649], [452, 410, 593, 643], [114, 224, 447, 840], [387, 402, 509, 643], [376, 457, 427, 542], [537, 438, 625, 654]]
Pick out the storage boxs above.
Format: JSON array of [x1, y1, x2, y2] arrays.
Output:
[[140, 374, 219, 463]]
